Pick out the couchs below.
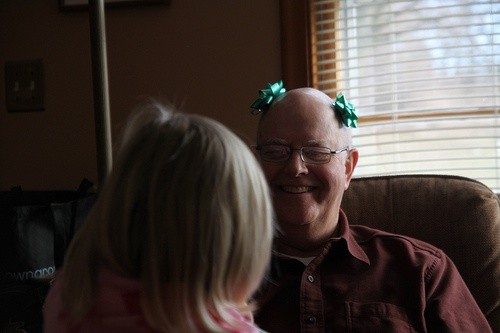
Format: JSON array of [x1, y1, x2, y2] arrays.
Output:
[[342, 175, 500, 333]]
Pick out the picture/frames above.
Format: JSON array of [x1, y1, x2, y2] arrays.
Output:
[[4, 59, 46, 113]]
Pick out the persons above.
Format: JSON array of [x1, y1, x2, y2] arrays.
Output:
[[42, 104, 276, 333], [248, 79, 495, 333]]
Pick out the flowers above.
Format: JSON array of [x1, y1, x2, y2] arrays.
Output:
[[250, 80, 286, 115], [333, 96, 358, 128]]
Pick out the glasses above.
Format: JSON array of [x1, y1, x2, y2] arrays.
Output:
[[256, 141, 349, 164]]
